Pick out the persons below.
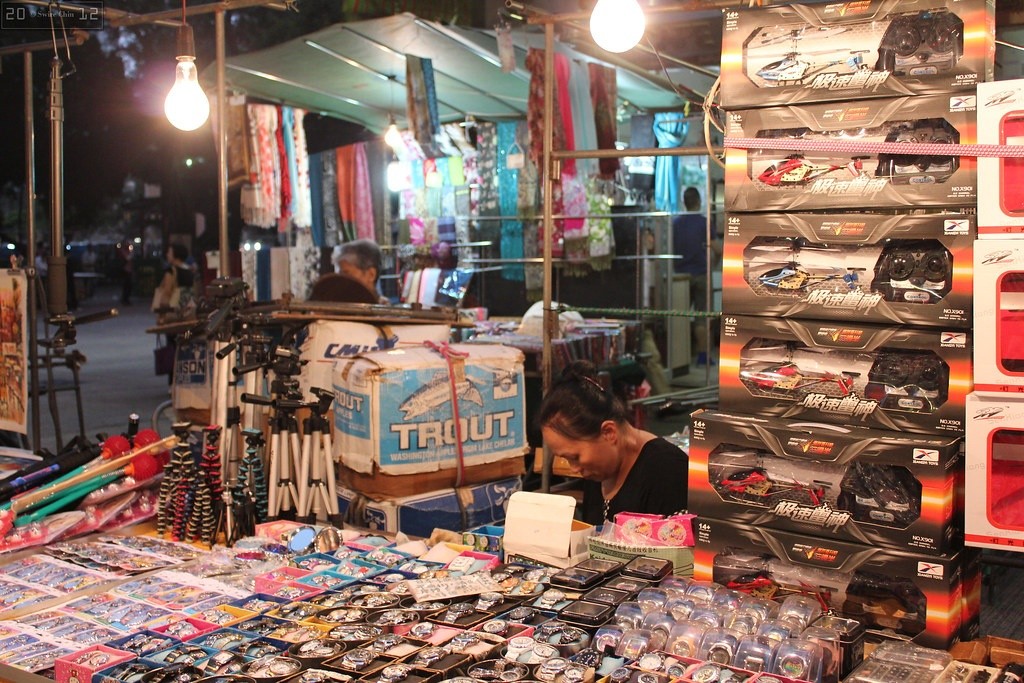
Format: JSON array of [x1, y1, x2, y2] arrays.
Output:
[[155, 241, 205, 401], [307, 238, 383, 304], [535, 357, 692, 526], [0, 233, 136, 307], [640, 186, 724, 395]]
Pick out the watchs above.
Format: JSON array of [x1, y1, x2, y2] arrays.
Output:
[[0, 531, 865, 683]]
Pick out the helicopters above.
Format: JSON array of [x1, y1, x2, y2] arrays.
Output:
[[756, 150, 874, 190], [723, 552, 838, 617], [749, 27, 870, 88], [707, 460, 832, 507], [760, 260, 866, 296], [741, 343, 863, 396]]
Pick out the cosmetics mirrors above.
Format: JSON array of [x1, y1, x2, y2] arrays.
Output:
[[287, 525, 317, 555], [313, 526, 343, 555]]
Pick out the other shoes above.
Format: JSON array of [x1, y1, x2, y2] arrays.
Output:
[[120, 299, 130, 305], [697, 357, 716, 368]]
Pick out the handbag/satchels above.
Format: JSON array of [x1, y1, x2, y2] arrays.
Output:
[[153, 324, 173, 376], [151, 266, 181, 313]]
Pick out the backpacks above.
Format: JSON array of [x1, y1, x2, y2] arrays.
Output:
[[106, 246, 130, 279]]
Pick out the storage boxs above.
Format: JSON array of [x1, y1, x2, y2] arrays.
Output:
[[174, 0, 1024, 649]]
